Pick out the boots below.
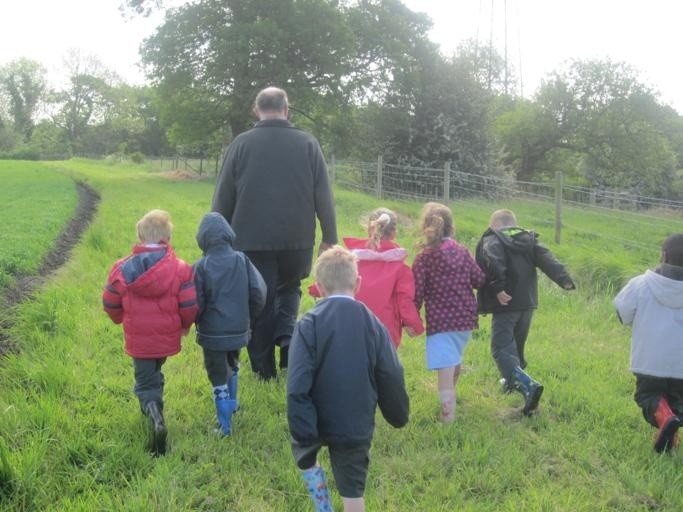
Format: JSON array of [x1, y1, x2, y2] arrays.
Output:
[[142, 402, 169, 454], [226, 374, 240, 413], [509, 366, 543, 416], [650, 396, 681, 454], [301, 467, 334, 512], [499, 360, 528, 394], [438, 389, 457, 428], [211, 386, 233, 437]]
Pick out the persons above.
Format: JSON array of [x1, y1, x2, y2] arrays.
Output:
[[400, 200, 486, 422], [102, 209, 197, 457], [307, 206, 423, 352], [286, 246, 409, 512], [475, 209, 575, 417], [209, 86, 338, 381], [611, 234, 682, 455], [189, 212, 265, 437]]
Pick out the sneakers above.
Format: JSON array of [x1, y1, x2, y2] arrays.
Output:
[[278, 344, 290, 372], [254, 370, 281, 386]]
